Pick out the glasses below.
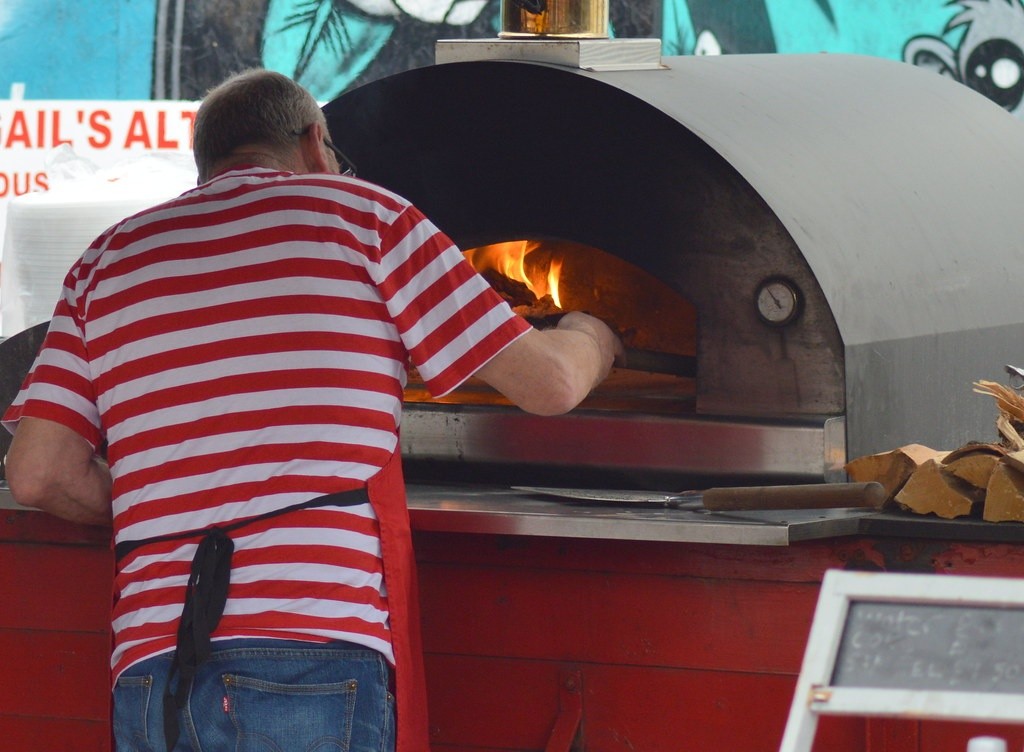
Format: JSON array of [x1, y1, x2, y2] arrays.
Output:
[[294, 128, 358, 177]]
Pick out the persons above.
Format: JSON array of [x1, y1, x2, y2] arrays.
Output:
[[0, 69, 617, 752]]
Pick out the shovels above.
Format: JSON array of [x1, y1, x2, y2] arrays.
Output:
[[511, 482, 888, 509]]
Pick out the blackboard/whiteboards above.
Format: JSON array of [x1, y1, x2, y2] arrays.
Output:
[[791, 567, 1024, 726]]
